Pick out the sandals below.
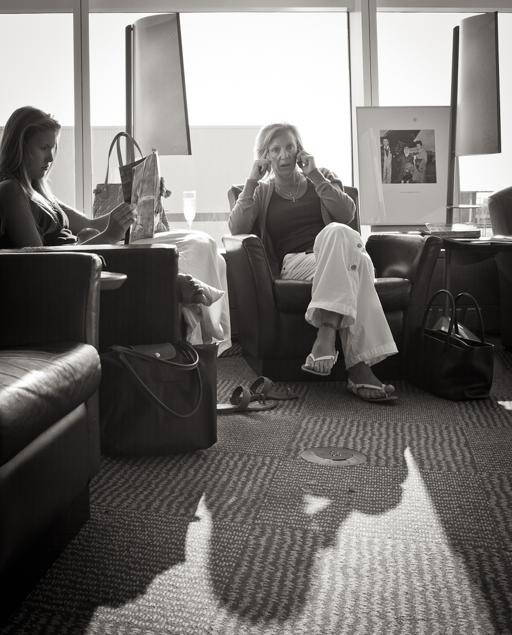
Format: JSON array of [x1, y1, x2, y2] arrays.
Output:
[[345, 378, 400, 404], [300, 350, 340, 376]]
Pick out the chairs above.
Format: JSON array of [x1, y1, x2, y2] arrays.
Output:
[[0, 246, 106, 575], [226, 184, 446, 383], [488, 183, 512, 377], [22, 243, 179, 354]]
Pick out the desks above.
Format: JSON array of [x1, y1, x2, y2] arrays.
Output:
[[417, 229, 512, 334]]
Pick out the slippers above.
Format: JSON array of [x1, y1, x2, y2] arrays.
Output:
[[231, 378, 298, 400], [216, 388, 278, 413]]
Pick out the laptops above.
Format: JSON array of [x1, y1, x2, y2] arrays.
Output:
[[423, 222, 481, 238]]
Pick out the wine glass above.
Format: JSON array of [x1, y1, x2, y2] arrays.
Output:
[[182, 190, 198, 229]]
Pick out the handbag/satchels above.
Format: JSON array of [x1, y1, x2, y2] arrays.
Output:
[[101, 342, 217, 455], [93, 184, 170, 233], [404, 290, 495, 400]]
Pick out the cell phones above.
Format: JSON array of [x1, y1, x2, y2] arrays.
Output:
[[299, 157, 308, 168]]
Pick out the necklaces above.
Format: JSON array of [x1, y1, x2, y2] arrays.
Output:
[[275, 172, 301, 203]]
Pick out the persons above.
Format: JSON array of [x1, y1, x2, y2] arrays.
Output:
[[380, 137, 428, 184], [0, 106, 207, 305], [228, 124, 400, 403]]
